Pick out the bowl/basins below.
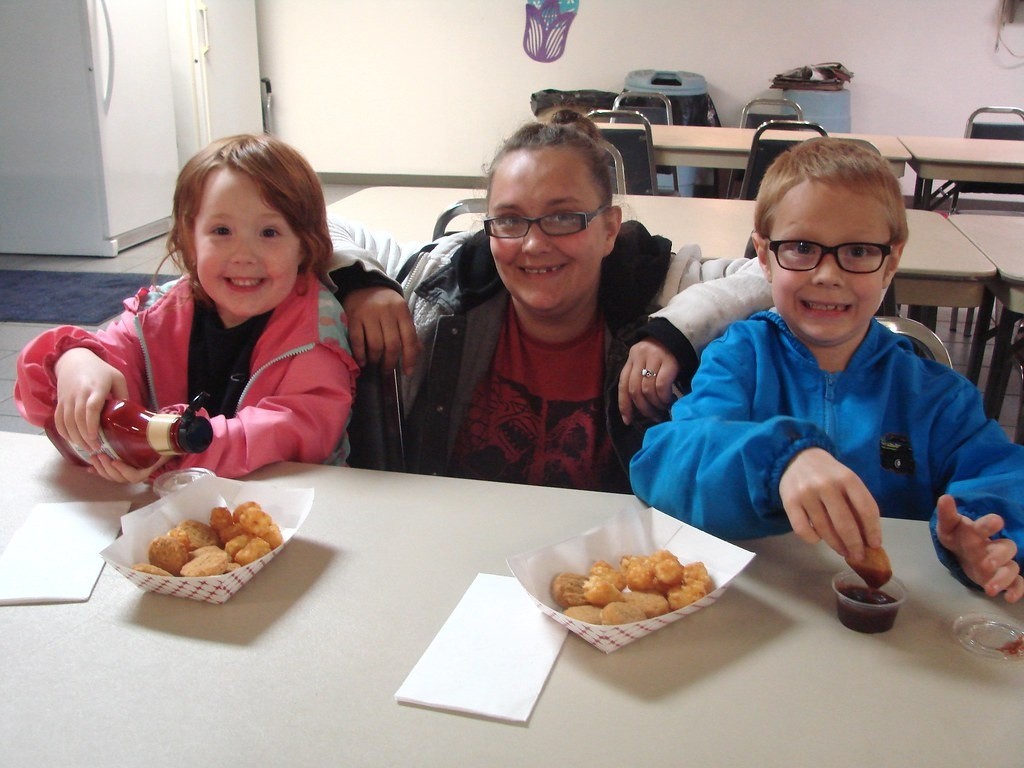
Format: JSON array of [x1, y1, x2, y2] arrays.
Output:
[[832, 570, 910, 634], [152, 467, 217, 499]]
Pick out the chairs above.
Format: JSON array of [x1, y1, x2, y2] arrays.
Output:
[[950, 106, 1024, 215], [346, 355, 408, 473], [585, 91, 832, 201], [432, 198, 490, 242], [871, 315, 954, 373]]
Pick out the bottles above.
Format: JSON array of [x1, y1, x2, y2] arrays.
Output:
[[44, 391, 214, 470]]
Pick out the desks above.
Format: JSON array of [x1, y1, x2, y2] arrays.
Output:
[[2, 429, 1023, 768], [0, 267, 180, 326], [896, 136, 1024, 212], [324, 188, 1023, 445], [592, 122, 913, 200]]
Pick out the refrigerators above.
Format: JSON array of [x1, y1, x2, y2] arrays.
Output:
[[0, 0, 264, 257]]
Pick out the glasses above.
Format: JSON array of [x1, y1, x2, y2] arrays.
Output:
[[483, 203, 610, 238], [764, 235, 895, 274]]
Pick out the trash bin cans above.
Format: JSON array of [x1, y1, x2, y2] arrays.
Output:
[[535, 89, 619, 122], [624, 69, 707, 198]]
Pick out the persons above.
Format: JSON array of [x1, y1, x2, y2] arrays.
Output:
[[14, 134, 361, 492], [318, 110, 772, 494], [626, 138, 1024, 602]]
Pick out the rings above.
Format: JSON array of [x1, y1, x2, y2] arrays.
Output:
[[641, 369, 656, 378]]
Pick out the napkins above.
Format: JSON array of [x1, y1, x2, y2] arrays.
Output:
[[2, 502, 133, 605], [393, 572, 571, 724]]
